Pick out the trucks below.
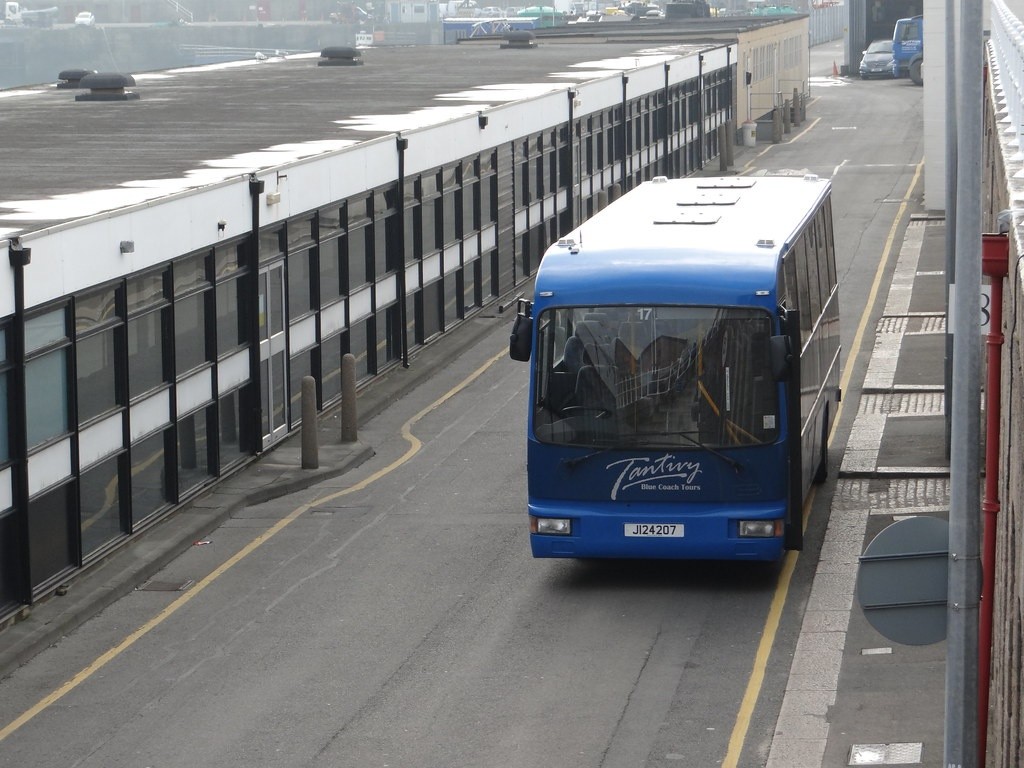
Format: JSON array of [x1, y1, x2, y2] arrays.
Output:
[[892, 15, 923, 86]]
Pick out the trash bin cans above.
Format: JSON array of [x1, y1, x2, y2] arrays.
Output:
[[742, 120, 757, 148]]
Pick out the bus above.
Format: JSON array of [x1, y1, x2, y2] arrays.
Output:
[[509, 173, 842, 565]]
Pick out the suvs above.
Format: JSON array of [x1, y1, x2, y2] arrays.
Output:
[[859, 40, 894, 79]]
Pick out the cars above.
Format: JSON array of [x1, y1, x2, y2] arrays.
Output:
[[75, 12, 95, 26]]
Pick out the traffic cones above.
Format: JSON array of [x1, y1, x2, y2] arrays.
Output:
[[832, 61, 839, 75]]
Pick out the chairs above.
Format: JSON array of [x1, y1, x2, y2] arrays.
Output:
[[560, 306, 696, 421]]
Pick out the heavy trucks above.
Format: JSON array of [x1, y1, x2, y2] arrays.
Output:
[[0, 0, 21, 25]]
